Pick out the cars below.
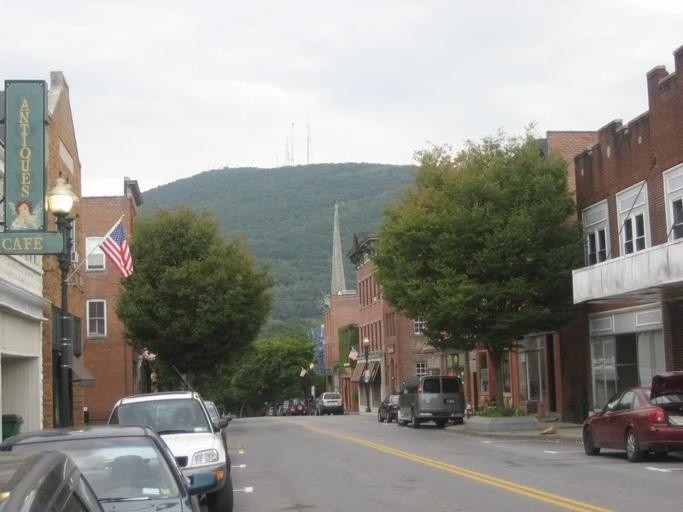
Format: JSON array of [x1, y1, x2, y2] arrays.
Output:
[[580, 368, 683, 461], [265, 391, 345, 417], [377, 393, 400, 423], [0, 362, 239, 511]]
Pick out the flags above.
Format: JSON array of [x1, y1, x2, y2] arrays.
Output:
[[347, 348, 359, 361], [95, 217, 134, 278], [297, 367, 306, 378]]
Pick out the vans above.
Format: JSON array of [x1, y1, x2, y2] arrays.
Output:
[[397, 373, 465, 429]]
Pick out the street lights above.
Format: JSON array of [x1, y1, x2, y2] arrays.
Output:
[[44, 170, 78, 423], [309, 362, 314, 406], [362, 336, 372, 412]]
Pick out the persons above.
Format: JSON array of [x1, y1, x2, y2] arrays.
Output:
[[8, 198, 40, 230]]
